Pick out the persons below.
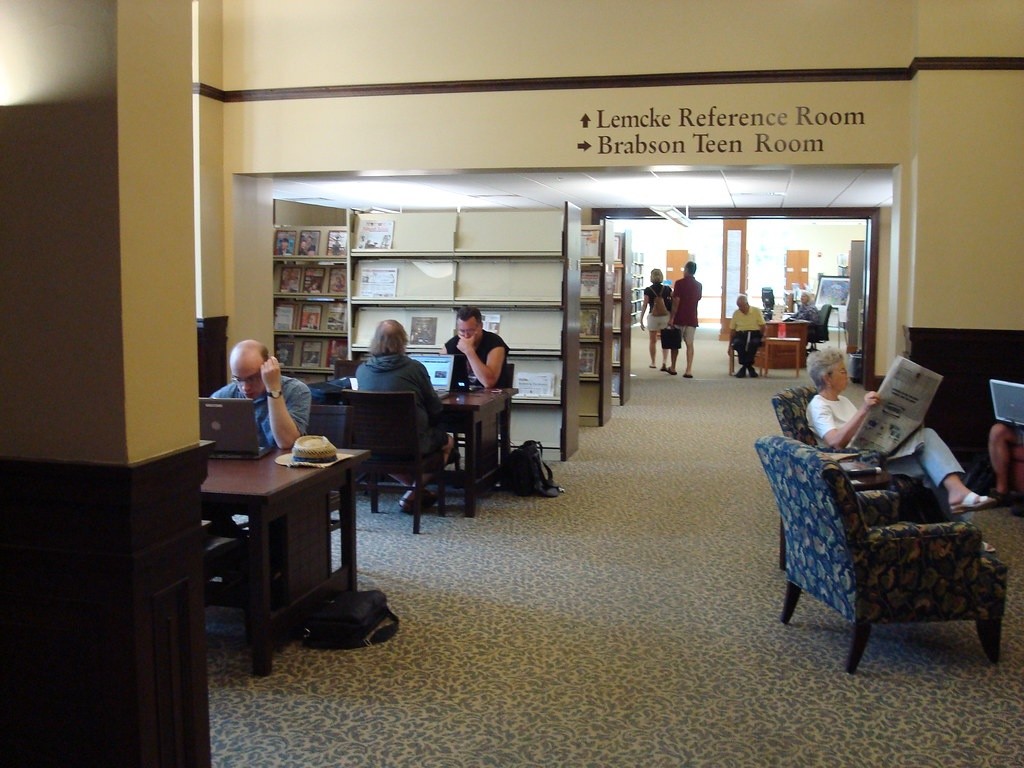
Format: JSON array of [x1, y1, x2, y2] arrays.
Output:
[[210, 340, 311, 582], [727, 294, 767, 378], [666, 261, 703, 378], [988, 422, 1024, 517], [640, 269, 672, 371], [805, 349, 996, 553], [444, 307, 508, 389], [355, 320, 456, 511], [791, 291, 825, 343]]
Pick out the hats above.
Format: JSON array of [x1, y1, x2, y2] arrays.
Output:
[[274, 435, 354, 468]]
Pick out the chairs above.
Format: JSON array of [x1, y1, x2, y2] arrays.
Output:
[[345, 390, 447, 533], [449, 363, 517, 485], [807, 303, 834, 353], [755, 386, 1007, 675]]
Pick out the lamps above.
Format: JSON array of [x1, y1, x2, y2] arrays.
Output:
[[648, 204, 692, 228]]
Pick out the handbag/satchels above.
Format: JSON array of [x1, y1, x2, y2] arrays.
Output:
[[891, 473, 945, 524], [660, 324, 681, 349], [294, 590, 398, 649]]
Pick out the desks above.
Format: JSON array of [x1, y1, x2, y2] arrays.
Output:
[[201, 443, 373, 674], [749, 316, 810, 368], [310, 387, 520, 518]]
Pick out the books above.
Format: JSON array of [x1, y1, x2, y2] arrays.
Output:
[[273, 229, 348, 368], [612, 234, 622, 397], [410, 317, 437, 345], [580, 229, 602, 378]]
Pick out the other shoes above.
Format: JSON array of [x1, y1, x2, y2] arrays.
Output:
[[748, 367, 758, 378], [735, 368, 746, 378]]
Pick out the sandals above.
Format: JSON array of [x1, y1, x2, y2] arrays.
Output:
[[397, 486, 439, 511]]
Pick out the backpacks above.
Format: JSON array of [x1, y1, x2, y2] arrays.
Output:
[[649, 285, 668, 316], [509, 440, 559, 497]]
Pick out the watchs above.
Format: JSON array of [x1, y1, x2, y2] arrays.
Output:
[[266, 391, 283, 399]]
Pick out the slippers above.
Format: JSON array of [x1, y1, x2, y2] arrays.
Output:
[[650, 366, 656, 368], [683, 373, 693, 378], [666, 367, 677, 375], [661, 368, 667, 371], [981, 541, 996, 553], [950, 492, 997, 514]]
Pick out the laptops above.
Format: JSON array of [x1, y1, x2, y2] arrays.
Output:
[[199, 397, 271, 459], [989, 379, 1024, 427], [407, 353, 484, 401]]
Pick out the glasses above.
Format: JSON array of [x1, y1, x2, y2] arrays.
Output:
[[232, 373, 261, 385], [457, 324, 478, 336]]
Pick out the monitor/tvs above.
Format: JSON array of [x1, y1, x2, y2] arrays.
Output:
[[763, 288, 775, 310]]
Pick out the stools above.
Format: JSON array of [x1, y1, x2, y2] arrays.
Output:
[[765, 337, 801, 379], [729, 341, 764, 376]]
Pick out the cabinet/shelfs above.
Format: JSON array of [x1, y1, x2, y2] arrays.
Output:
[[631, 251, 647, 325], [784, 249, 810, 290], [666, 249, 696, 289], [344, 202, 585, 464], [270, 214, 616, 427], [610, 231, 634, 407]]
